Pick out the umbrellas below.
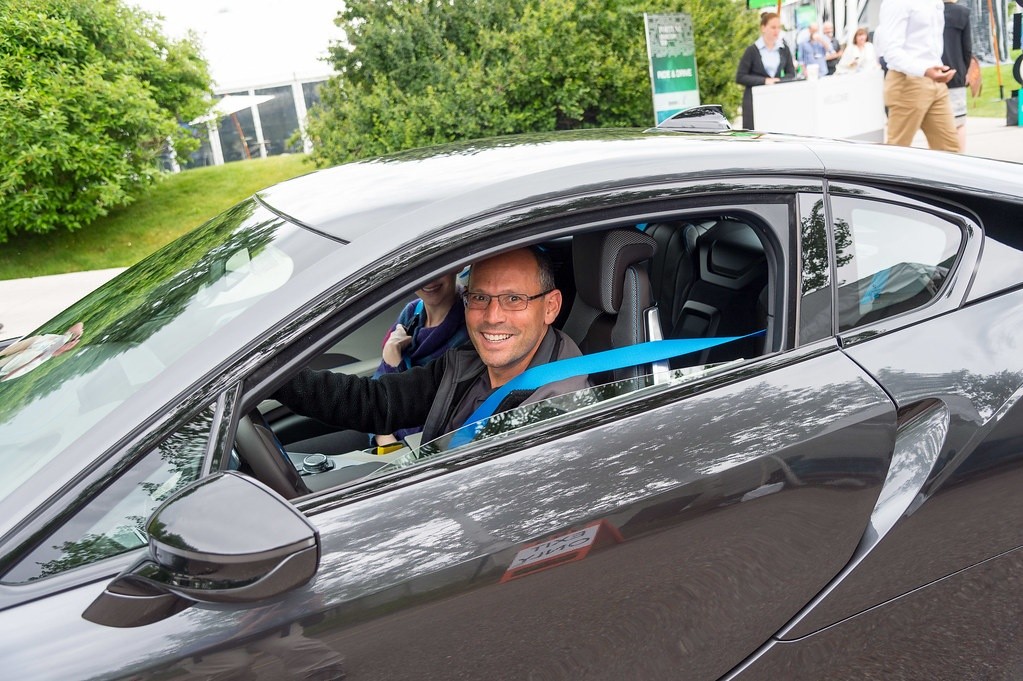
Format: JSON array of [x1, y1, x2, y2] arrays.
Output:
[[188, 94, 275, 160]]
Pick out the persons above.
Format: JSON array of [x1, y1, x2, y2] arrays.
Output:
[[877, 0, 964, 155], [873, 25, 889, 120], [0, 321, 84, 384], [171, 583, 349, 681], [264, 243, 606, 461], [820, 20, 843, 76], [941, 0, 973, 150], [797, 20, 834, 80], [837, 26, 877, 73], [279, 265, 471, 458], [734, 11, 796, 131]]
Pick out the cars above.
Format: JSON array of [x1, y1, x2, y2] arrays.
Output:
[[1, 103, 1023, 681]]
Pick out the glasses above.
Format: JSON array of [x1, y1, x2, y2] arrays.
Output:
[[460, 289, 555, 311]]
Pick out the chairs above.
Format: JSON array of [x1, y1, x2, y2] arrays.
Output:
[[556, 222, 775, 397]]
